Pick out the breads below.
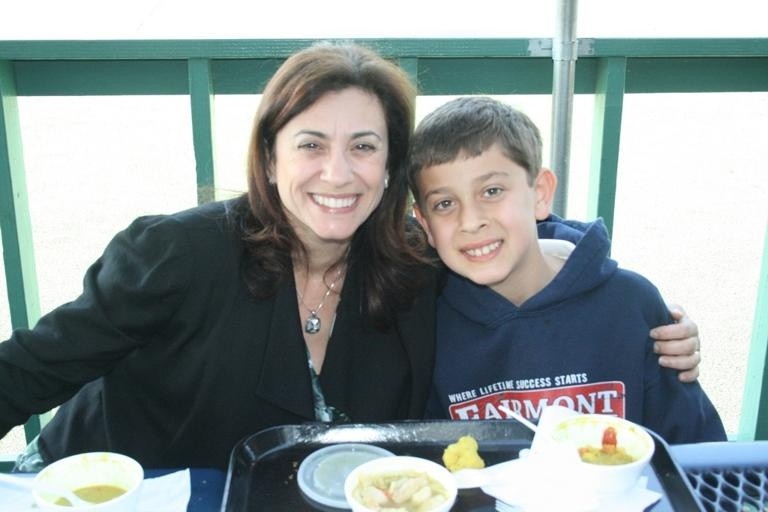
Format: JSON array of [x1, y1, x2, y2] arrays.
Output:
[[442, 435, 485, 472]]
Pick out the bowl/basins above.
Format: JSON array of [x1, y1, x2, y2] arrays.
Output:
[[341, 453, 462, 512], [30, 450, 146, 511], [547, 411, 657, 497]]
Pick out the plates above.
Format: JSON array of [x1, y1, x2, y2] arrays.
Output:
[[474, 452, 666, 511]]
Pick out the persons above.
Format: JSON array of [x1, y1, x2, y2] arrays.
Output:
[[0, 43, 702, 473], [405, 96, 741, 512]]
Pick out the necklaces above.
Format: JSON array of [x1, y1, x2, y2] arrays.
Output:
[[296, 269, 343, 335]]
